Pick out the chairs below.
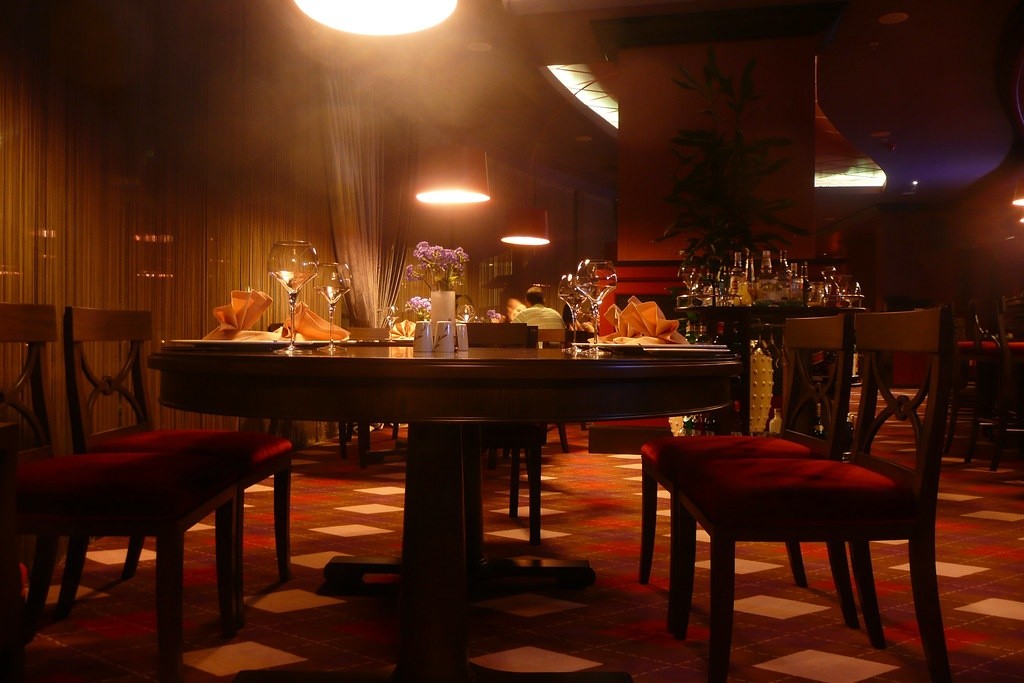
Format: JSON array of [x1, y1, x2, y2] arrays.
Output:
[[638, 304, 955, 683], [0, 304, 292, 683], [338, 323, 599, 545]]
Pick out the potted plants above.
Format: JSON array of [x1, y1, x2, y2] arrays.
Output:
[[653, 44, 813, 439]]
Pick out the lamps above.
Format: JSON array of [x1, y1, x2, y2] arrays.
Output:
[[500, 173, 550, 245], [414, 84, 490, 203]]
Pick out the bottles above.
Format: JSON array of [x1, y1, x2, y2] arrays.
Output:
[[668, 413, 720, 436], [703, 248, 812, 307], [684, 320, 744, 353], [843, 412, 857, 452], [730, 400, 744, 435], [813, 403, 826, 439], [769, 408, 783, 433], [810, 350, 838, 384]]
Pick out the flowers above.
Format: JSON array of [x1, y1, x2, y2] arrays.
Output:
[[406, 242, 469, 291], [405, 296, 431, 322], [486, 309, 501, 323]]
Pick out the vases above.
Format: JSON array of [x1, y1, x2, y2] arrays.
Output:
[[432, 291, 458, 351]]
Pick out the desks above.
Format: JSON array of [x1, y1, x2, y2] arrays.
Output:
[[674, 306, 865, 437], [147, 337, 748, 683]]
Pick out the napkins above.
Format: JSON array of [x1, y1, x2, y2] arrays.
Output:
[[390, 319, 416, 337], [588, 296, 690, 344], [201, 290, 281, 340], [281, 301, 351, 341]]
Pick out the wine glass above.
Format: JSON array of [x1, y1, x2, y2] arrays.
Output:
[[677, 265, 702, 308], [575, 259, 617, 357], [557, 273, 592, 354], [267, 240, 319, 354], [312, 262, 353, 351]]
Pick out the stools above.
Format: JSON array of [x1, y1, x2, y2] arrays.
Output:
[[946, 293, 1024, 471]]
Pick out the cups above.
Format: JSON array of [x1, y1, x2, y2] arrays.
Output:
[[809, 282, 823, 306], [456, 324, 469, 351], [821, 266, 860, 306], [413, 321, 433, 351], [434, 321, 454, 352]]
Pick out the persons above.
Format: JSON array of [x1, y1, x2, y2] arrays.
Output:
[[828, 229, 860, 273], [505, 287, 594, 333]]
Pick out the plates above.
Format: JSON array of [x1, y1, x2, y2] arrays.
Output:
[[391, 338, 414, 341], [570, 343, 727, 348], [170, 340, 291, 351], [643, 348, 730, 353], [293, 340, 357, 348]]
